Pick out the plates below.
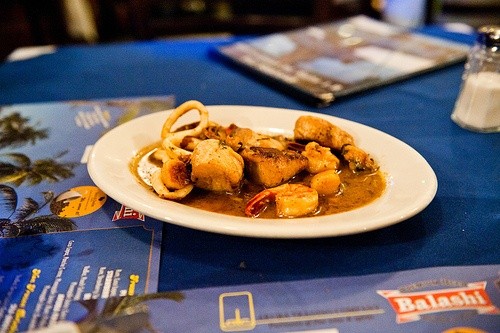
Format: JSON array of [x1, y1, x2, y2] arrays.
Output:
[[88, 105, 438, 238]]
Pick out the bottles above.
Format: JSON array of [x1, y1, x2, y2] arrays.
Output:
[[449, 25, 500, 132]]
